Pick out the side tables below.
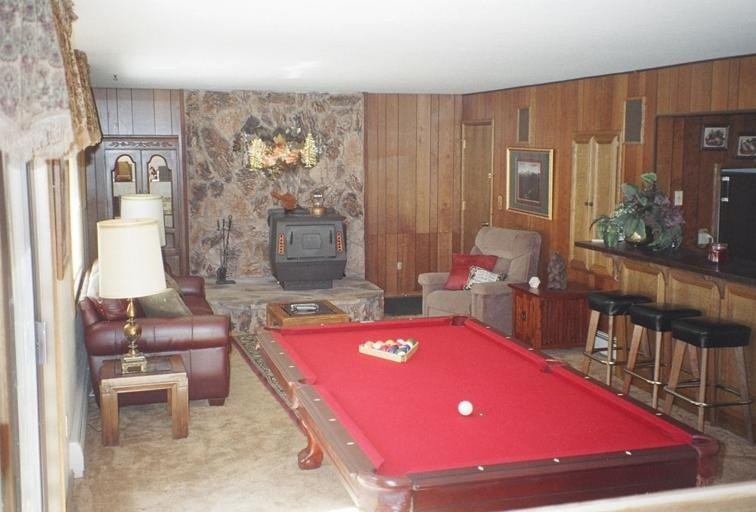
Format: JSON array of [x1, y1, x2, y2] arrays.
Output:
[[97, 355, 191, 445], [508, 283, 603, 351]]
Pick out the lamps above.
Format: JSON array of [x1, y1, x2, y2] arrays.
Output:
[[97, 219, 167, 373], [120, 194, 166, 248]]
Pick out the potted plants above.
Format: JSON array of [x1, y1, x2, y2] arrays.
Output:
[[587, 172, 687, 252]]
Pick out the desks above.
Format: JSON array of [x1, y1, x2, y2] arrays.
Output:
[[255, 313, 726, 512]]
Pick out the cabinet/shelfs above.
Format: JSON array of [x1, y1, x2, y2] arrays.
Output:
[[601, 252, 752, 442]]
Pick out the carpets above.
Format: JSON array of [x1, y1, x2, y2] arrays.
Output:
[[231, 334, 306, 438]]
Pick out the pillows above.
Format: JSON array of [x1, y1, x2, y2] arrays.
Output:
[[444, 253, 497, 290], [138, 288, 195, 317], [86, 259, 131, 321], [462, 264, 507, 290], [167, 280, 185, 298]]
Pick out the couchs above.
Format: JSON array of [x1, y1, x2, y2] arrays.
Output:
[[76, 259, 231, 410]]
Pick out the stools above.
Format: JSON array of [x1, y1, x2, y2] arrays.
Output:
[[582, 292, 653, 386]]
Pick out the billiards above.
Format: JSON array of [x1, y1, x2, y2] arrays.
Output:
[[363, 340, 416, 359], [459, 401, 471, 411]]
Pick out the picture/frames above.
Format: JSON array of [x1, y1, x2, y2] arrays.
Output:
[[505, 146, 557, 221], [734, 133, 756, 160], [699, 123, 729, 151]]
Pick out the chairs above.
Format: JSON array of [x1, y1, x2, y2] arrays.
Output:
[[417, 227, 542, 337]]
